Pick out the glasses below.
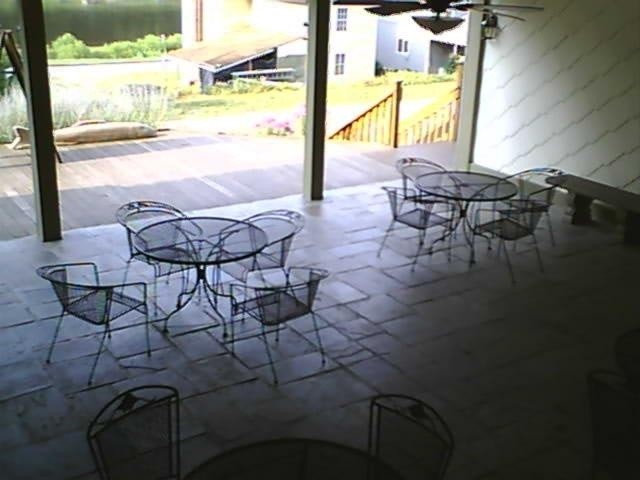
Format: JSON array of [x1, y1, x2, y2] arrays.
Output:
[[479, 12, 501, 44], [412, 11, 466, 37]]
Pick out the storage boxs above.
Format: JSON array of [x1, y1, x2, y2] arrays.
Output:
[[547, 175, 640, 243]]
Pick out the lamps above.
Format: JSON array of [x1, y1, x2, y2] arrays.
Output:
[[397, 157, 461, 246], [86, 385, 182, 479], [469, 200, 542, 282], [218, 210, 306, 320], [491, 168, 566, 255], [230, 267, 329, 384], [115, 201, 203, 311], [368, 396, 455, 480], [38, 263, 150, 383], [376, 186, 454, 272]]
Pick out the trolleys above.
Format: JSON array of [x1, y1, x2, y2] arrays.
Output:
[[332, 0, 546, 37]]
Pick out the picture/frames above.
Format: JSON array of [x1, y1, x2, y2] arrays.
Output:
[[414, 173, 517, 265], [137, 218, 267, 337], [183, 439, 404, 479]]
